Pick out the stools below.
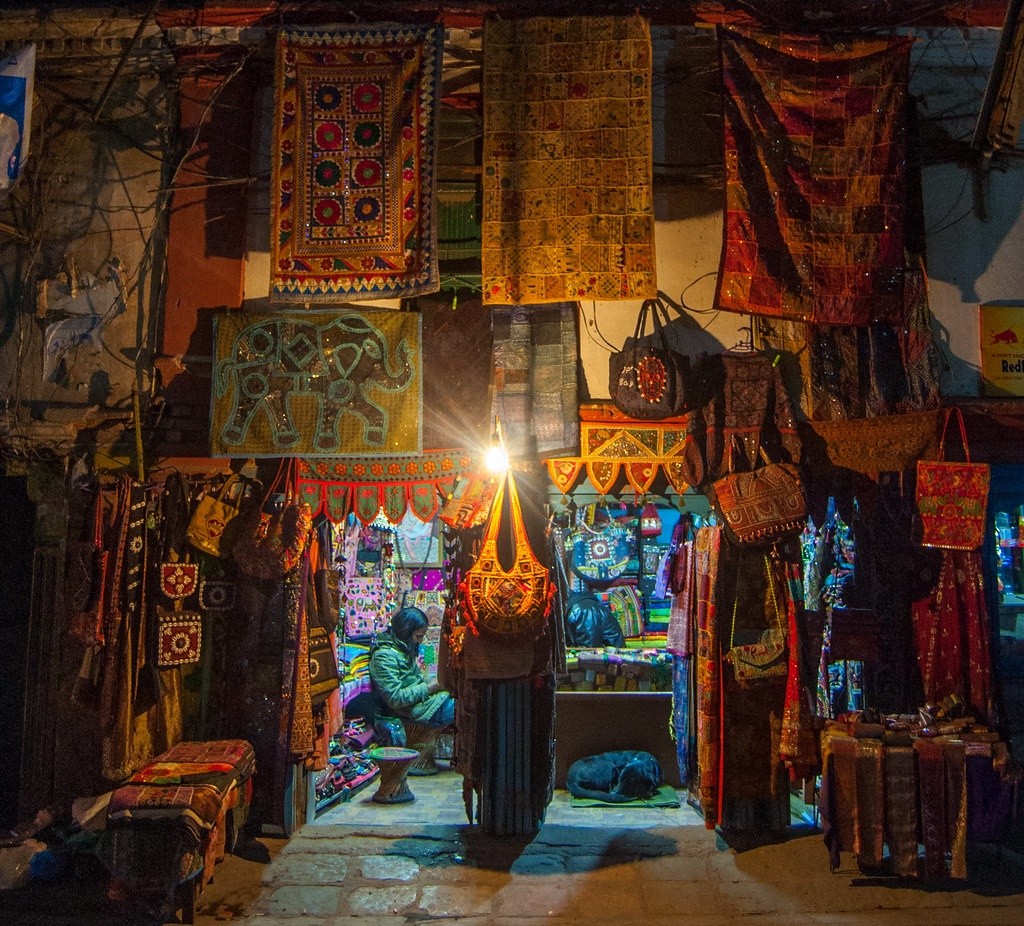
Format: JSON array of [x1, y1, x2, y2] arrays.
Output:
[[400, 718, 450, 776], [368, 747, 420, 804]]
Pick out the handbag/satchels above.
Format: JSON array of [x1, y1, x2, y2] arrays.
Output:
[[185, 472, 248, 561], [608, 300, 687, 420], [732, 642, 787, 678], [655, 525, 680, 600], [343, 577, 387, 638], [725, 626, 787, 690], [571, 504, 630, 582], [703, 442, 808, 552], [832, 608, 875, 661], [457, 472, 554, 647], [233, 454, 314, 579], [671, 523, 686, 593], [317, 569, 340, 634], [307, 629, 340, 705], [912, 405, 991, 550], [401, 568, 450, 610]]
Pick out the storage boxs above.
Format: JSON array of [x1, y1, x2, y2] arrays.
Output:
[[979, 305, 1023, 402]]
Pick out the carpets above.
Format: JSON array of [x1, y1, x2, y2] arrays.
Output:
[[33, 3, 1024, 926]]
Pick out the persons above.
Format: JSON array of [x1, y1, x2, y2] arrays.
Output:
[[369, 607, 455, 726], [564, 588, 627, 648]]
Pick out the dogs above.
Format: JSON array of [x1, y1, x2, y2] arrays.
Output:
[[566, 749, 664, 803]]
